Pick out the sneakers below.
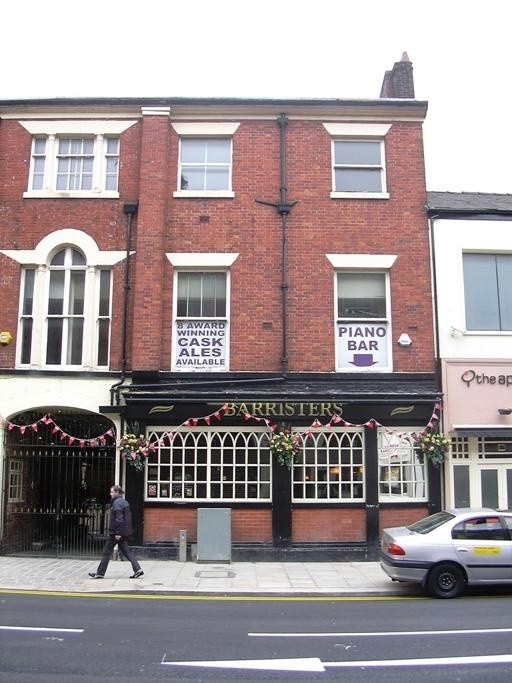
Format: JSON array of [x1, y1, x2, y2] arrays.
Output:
[[130, 569, 144, 579], [89, 573, 104, 579]]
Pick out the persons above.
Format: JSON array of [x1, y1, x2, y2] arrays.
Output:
[[89, 484, 144, 579]]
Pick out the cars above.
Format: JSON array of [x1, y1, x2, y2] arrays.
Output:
[[377, 507, 512, 598]]
